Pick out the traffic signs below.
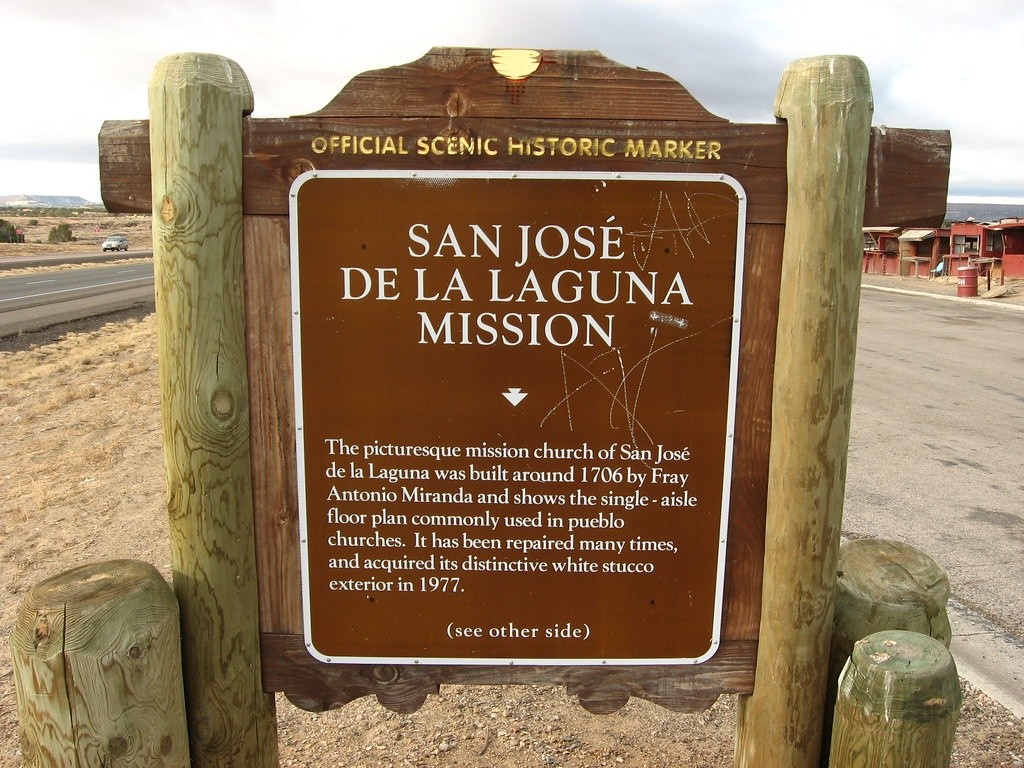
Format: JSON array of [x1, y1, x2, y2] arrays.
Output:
[[286, 169, 747, 662]]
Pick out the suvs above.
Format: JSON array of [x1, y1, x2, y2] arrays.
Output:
[[101, 236, 128, 252]]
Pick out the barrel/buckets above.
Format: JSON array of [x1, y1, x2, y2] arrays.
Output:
[[958, 267, 978, 296]]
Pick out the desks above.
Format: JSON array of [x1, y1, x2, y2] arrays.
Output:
[[898, 256, 931, 279]]
